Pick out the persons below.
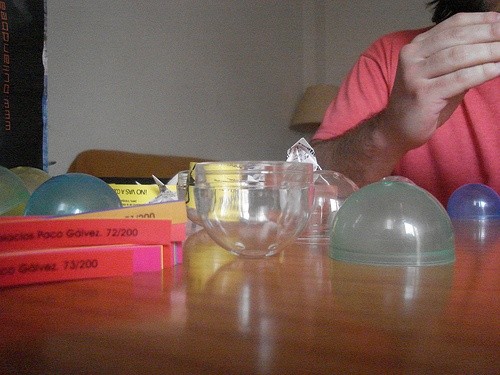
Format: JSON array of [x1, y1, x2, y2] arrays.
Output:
[[308, 0, 500, 208]]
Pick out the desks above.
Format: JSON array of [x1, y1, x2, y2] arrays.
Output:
[[0, 223, 500, 375]]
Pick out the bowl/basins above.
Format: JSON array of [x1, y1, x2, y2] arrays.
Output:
[[295, 170, 359, 240], [195, 161, 314, 259], [383, 176, 416, 185], [178, 170, 204, 227]]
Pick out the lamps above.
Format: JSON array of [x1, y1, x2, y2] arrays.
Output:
[[289, 84, 340, 132]]
